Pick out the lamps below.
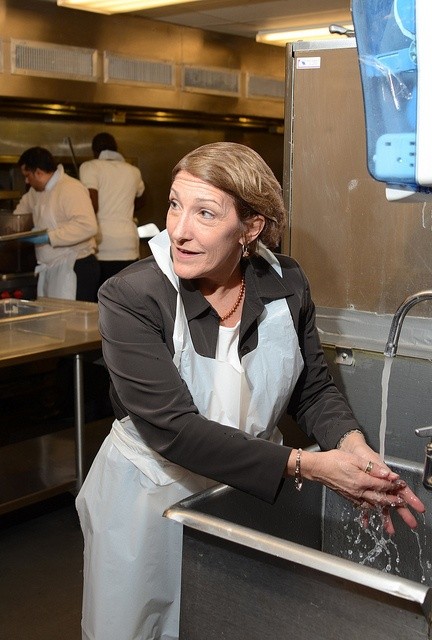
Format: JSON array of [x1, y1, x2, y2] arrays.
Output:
[[254, 20, 355, 43]]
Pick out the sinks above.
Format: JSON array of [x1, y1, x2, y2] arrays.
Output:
[[161, 440, 431, 639]]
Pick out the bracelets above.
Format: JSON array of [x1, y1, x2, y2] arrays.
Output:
[[294, 448, 303, 495]]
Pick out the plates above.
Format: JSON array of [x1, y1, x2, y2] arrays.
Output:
[[0, 229, 47, 241]]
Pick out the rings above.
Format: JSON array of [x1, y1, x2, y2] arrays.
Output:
[[365, 460, 375, 475]]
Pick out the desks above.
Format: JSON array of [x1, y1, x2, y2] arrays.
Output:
[[1, 297, 101, 516]]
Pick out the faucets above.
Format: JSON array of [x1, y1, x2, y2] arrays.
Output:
[[383, 291, 431, 490]]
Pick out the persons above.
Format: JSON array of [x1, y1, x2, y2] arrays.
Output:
[[78, 133, 145, 299], [7, 146, 98, 302], [76, 141, 422, 639]]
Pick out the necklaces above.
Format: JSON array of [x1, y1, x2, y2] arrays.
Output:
[[220, 276, 245, 321]]
